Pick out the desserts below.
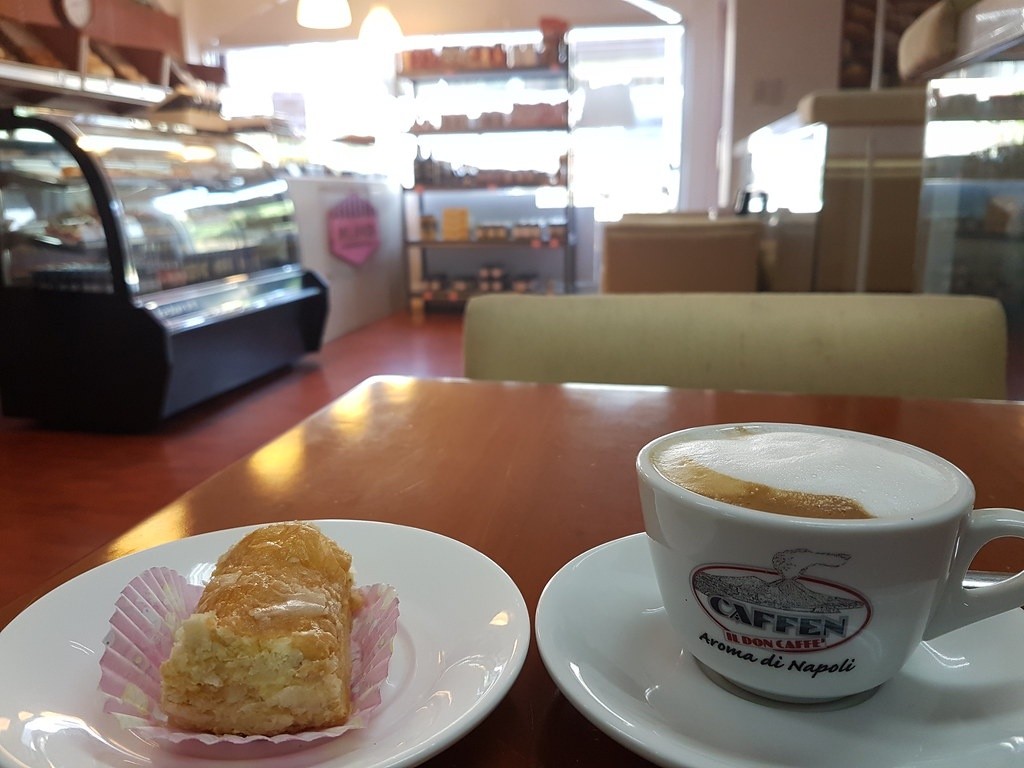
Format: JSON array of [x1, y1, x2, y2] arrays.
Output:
[[157, 521, 354, 736]]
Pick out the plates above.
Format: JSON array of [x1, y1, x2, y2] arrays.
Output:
[[533, 528, 1023, 768], [1, 518, 531, 767]]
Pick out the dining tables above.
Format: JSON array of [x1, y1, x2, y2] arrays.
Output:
[[0, 375, 1024, 768]]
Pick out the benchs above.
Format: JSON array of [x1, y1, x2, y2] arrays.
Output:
[[458, 295, 1008, 399]]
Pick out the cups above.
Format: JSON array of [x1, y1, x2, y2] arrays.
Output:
[[637, 418, 1023, 709]]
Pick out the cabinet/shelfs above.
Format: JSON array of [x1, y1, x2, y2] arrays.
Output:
[[390, 19, 578, 324]]
